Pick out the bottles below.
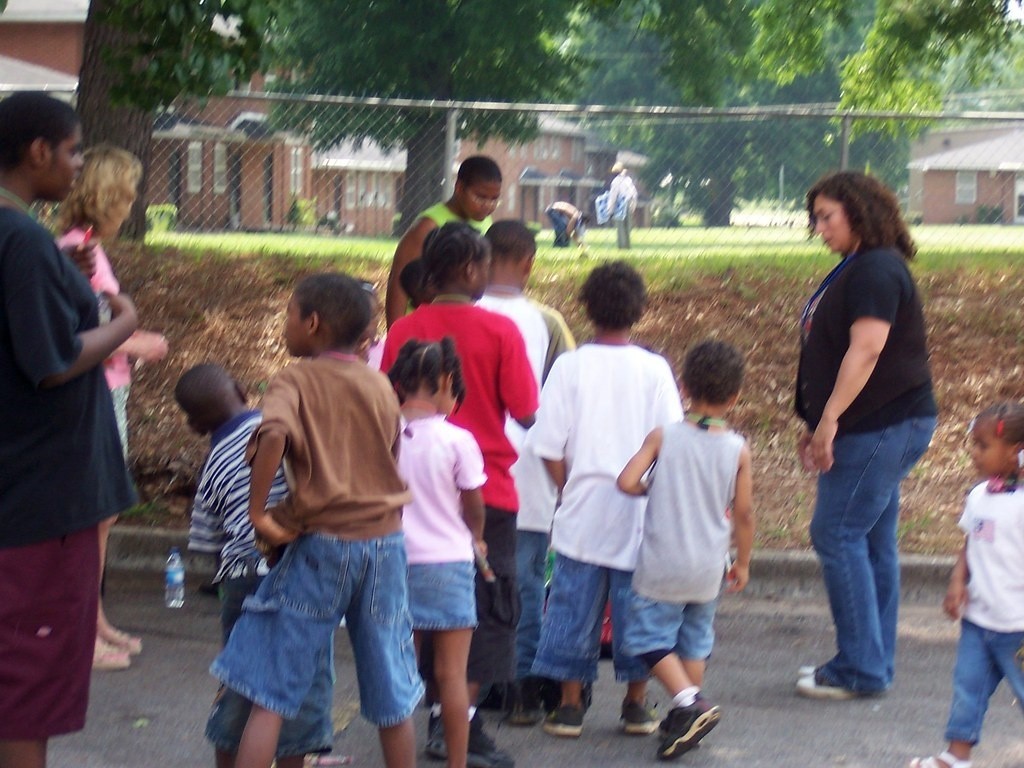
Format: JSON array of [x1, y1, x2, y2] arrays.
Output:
[[164, 546, 185, 609]]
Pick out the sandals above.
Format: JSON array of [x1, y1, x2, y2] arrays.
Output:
[[904, 751, 973, 768]]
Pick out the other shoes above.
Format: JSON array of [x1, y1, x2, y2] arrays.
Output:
[[622, 691, 662, 733], [427, 708, 515, 768], [797, 664, 859, 700], [509, 684, 547, 724], [544, 689, 591, 736], [90, 637, 131, 671], [99, 626, 142, 654], [276, 752, 355, 768]]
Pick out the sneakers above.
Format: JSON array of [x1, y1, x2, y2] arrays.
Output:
[[658, 692, 720, 758]]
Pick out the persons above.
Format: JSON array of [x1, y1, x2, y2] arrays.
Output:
[[793, 171, 937, 702], [615, 342, 757, 759], [384, 219, 538, 767], [610, 162, 638, 248], [55, 145, 170, 670], [545, 202, 591, 246], [387, 333, 488, 766], [0, 90, 139, 768], [531, 261, 684, 737], [912, 403, 1024, 768], [386, 155, 502, 328], [476, 217, 577, 726], [208, 272, 426, 768], [176, 363, 352, 768]]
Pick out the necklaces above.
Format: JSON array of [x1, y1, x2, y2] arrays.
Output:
[[686, 414, 727, 428], [430, 294, 470, 302], [484, 285, 520, 295]]
